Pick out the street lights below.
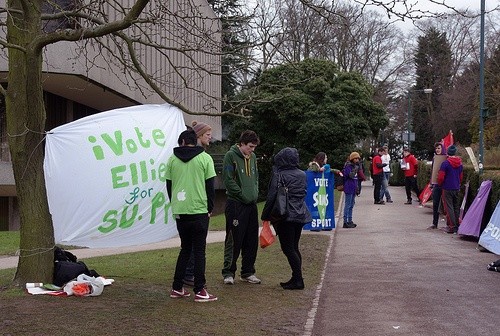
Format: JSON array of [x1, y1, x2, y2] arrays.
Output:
[[407, 88, 433, 151]]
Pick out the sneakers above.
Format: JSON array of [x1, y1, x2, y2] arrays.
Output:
[[224, 277, 233, 284], [240, 275, 261, 284], [183, 276, 207, 286], [170, 287, 190, 297], [194, 288, 217, 302]]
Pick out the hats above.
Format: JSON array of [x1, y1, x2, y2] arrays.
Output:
[[192, 121, 211, 137], [350, 152, 360, 160], [447, 145, 456, 155]]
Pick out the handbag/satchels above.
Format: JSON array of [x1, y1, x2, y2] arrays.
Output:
[[266, 187, 286, 221], [54, 248, 89, 287]]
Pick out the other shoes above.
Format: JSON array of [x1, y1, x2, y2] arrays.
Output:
[[343, 222, 357, 228], [404, 201, 412, 204], [280, 278, 305, 290], [387, 200, 393, 203], [374, 201, 385, 205], [426, 225, 438, 229], [447, 228, 458, 234]]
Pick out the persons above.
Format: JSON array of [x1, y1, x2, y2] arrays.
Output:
[[165, 129, 218, 302], [182, 120, 211, 289], [221, 130, 262, 285], [402, 149, 422, 204], [261, 147, 313, 290], [370, 147, 380, 186], [379, 147, 394, 203], [372, 147, 388, 204], [343, 152, 361, 228], [438, 145, 463, 233], [427, 141, 450, 229], [307, 151, 343, 176]]
